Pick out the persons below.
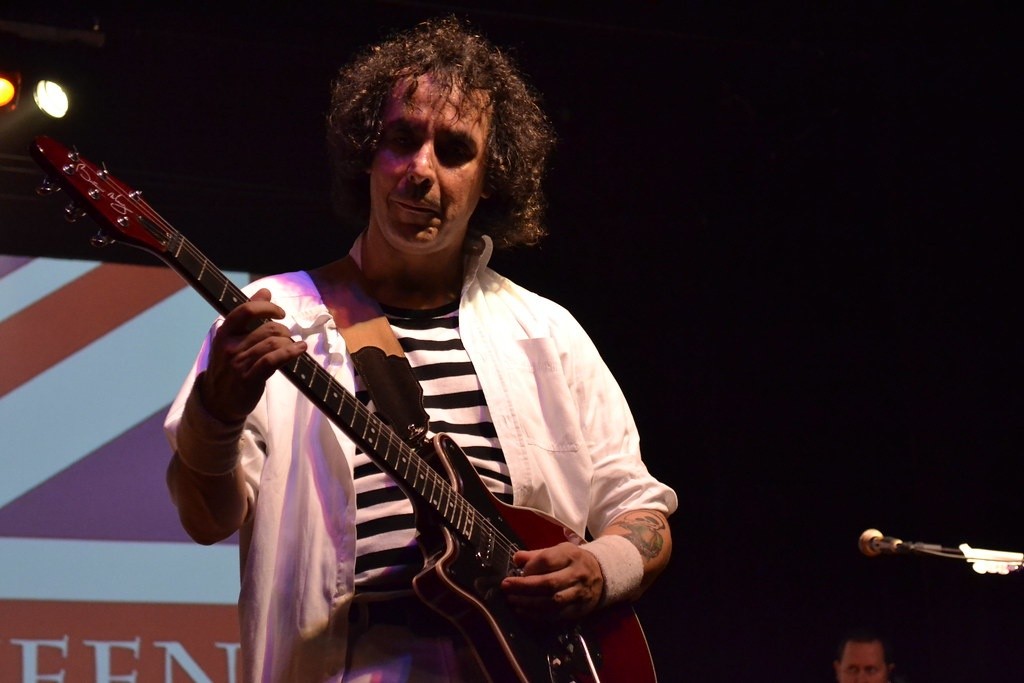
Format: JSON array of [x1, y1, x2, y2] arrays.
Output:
[[830, 628, 899, 681], [164, 20, 680, 683]]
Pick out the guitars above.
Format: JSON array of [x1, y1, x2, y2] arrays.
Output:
[[0, 82, 666, 683]]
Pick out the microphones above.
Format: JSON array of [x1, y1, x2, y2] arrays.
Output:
[[858, 528, 901, 557]]
